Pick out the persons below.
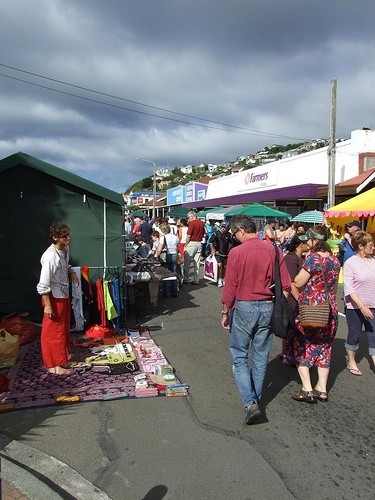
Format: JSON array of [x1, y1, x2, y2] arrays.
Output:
[[340, 220, 362, 262], [205, 221, 220, 237], [342, 231, 375, 376], [263, 219, 304, 246], [37, 223, 79, 375], [279, 233, 309, 368], [125, 216, 188, 267], [209, 222, 234, 287], [154, 224, 180, 297], [183, 210, 205, 285], [221, 214, 292, 425], [281, 225, 341, 403]]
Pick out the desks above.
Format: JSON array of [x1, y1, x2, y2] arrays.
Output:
[[125, 256, 177, 310]]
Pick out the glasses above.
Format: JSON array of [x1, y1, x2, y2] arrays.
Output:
[[231, 225, 247, 239]]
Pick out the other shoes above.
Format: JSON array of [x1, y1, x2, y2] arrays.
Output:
[[164, 296, 169, 298], [244, 403, 262, 425], [171, 295, 178, 298], [217, 282, 223, 288], [191, 282, 198, 285]]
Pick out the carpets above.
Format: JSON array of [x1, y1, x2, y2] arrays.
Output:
[[0, 323, 189, 413]]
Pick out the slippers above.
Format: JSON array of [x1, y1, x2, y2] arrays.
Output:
[[345, 365, 362, 376]]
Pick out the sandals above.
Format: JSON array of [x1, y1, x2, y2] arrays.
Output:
[[292, 390, 315, 402], [313, 389, 328, 402]]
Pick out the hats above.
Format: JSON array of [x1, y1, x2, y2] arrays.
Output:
[[270, 220, 278, 223], [298, 228, 327, 241], [221, 222, 228, 227], [345, 220, 362, 229]]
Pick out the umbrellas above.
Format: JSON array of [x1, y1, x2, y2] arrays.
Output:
[[130, 210, 147, 216], [290, 210, 323, 225], [167, 207, 190, 217], [225, 202, 290, 216]]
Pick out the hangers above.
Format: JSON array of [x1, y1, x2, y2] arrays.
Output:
[[96, 265, 123, 283]]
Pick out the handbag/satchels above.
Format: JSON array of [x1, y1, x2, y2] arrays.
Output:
[[203, 254, 218, 283], [158, 236, 167, 263], [298, 304, 329, 328], [270, 296, 294, 339]]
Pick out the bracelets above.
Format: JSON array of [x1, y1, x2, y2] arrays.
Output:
[[361, 305, 365, 309], [222, 311, 228, 314]]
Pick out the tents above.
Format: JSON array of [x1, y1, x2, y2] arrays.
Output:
[[323, 187, 375, 239], [0, 151, 125, 303]]
